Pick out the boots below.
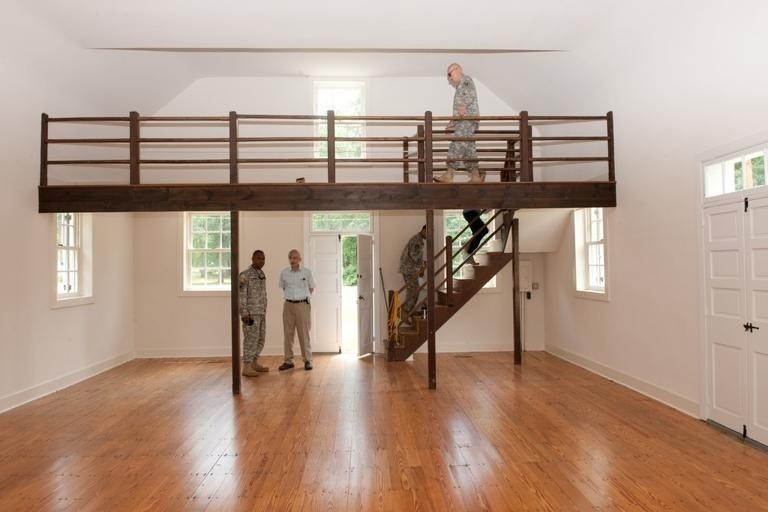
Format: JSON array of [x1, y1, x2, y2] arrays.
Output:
[[465, 168, 482, 184], [432, 168, 455, 183], [397, 306, 411, 325], [242, 361, 270, 376]]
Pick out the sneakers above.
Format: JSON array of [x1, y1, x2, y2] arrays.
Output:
[[461, 239, 479, 265]]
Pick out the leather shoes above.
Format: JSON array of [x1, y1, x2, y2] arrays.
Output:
[[305, 361, 312, 370], [276, 362, 295, 371]]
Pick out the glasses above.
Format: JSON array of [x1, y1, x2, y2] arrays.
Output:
[[448, 68, 458, 77]]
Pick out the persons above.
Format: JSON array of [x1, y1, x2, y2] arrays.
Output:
[[279, 249, 315, 371], [463, 168, 489, 265], [433, 63, 482, 185], [239, 250, 269, 377], [398, 224, 426, 325]]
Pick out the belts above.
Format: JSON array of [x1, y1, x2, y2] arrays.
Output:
[[285, 298, 309, 304]]
